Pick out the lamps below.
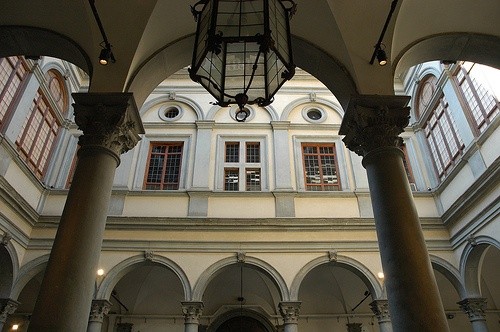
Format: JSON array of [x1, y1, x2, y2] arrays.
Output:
[[98, 40, 114, 65], [375, 42, 388, 66], [184, 0, 295, 107]]
